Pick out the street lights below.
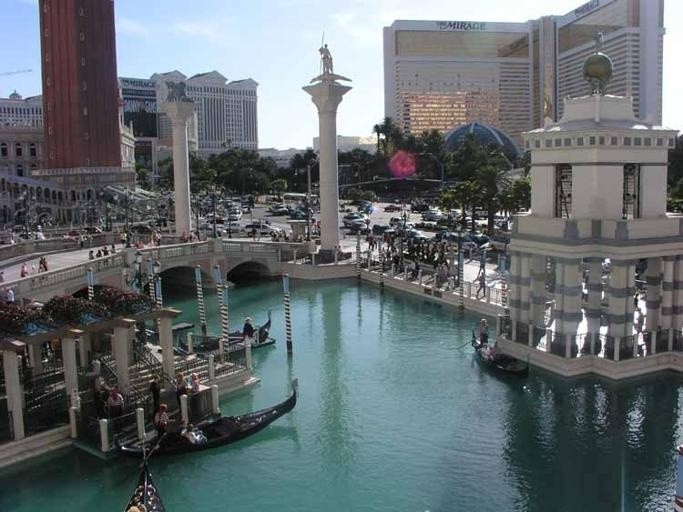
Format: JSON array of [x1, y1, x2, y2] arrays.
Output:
[[306, 193, 311, 241], [122, 251, 162, 295]]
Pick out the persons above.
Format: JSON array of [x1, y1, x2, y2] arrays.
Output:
[[319, 44, 332, 73], [393, 195, 428, 213], [633, 284, 646, 335], [53, 229, 162, 260], [366, 231, 456, 290], [476, 272, 486, 299], [477, 250, 486, 281], [243, 317, 258, 337], [21, 256, 48, 277], [0, 271, 5, 283], [180, 231, 199, 243], [89, 342, 125, 435], [148, 373, 209, 451], [477, 318, 502, 363]]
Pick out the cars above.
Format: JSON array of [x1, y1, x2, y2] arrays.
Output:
[[204, 196, 282, 237], [268, 193, 317, 218], [343, 199, 513, 251]]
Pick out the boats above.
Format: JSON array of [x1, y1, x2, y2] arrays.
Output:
[[472, 332, 528, 375], [179, 310, 271, 353], [121, 390, 296, 512]]
